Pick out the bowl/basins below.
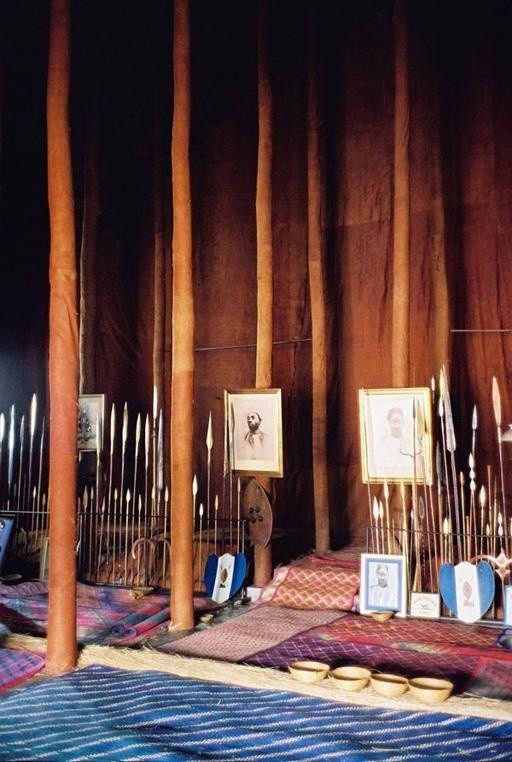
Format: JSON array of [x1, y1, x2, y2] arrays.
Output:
[[370, 611, 394, 623], [329, 665, 371, 691], [407, 677, 455, 705], [1, 573, 23, 585], [199, 613, 213, 623], [287, 660, 329, 684], [370, 673, 408, 697]]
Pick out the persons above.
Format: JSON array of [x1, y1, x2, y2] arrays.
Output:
[[78, 402, 97, 442], [380, 405, 412, 461], [244, 410, 266, 451], [370, 564, 395, 606], [459, 580, 474, 608]]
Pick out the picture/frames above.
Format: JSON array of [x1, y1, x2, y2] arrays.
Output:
[[221, 387, 287, 481], [410, 591, 443, 621], [355, 386, 438, 487], [358, 552, 408, 615], [74, 393, 107, 453]]
